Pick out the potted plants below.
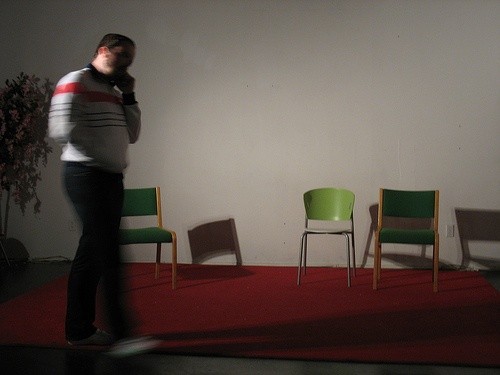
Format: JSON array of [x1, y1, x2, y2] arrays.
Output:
[[0, 71, 57, 271]]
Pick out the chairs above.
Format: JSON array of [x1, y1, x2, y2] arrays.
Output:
[[297, 187, 359, 287], [373, 187, 439, 293], [120, 186, 177, 288]]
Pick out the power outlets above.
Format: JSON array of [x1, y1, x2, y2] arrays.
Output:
[[445, 225, 454, 239]]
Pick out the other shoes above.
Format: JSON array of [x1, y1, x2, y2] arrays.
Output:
[[68, 329, 112, 345]]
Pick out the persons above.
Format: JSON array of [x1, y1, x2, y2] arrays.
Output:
[[48, 33, 161, 357]]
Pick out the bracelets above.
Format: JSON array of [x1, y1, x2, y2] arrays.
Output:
[[121, 92, 136, 103]]
